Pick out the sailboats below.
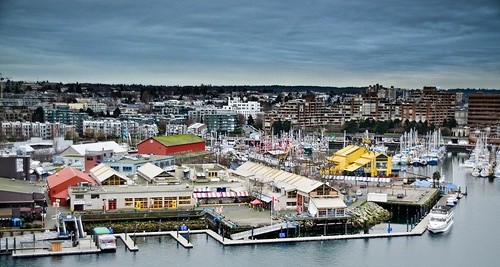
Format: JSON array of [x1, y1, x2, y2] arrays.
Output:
[[233, 119, 500, 184]]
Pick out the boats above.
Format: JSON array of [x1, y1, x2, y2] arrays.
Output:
[[426, 200, 453, 234], [92, 227, 117, 252]]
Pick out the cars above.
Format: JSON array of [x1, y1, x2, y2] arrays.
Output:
[[347, 183, 352, 187], [360, 184, 366, 189], [397, 193, 404, 199], [341, 188, 363, 205]]
